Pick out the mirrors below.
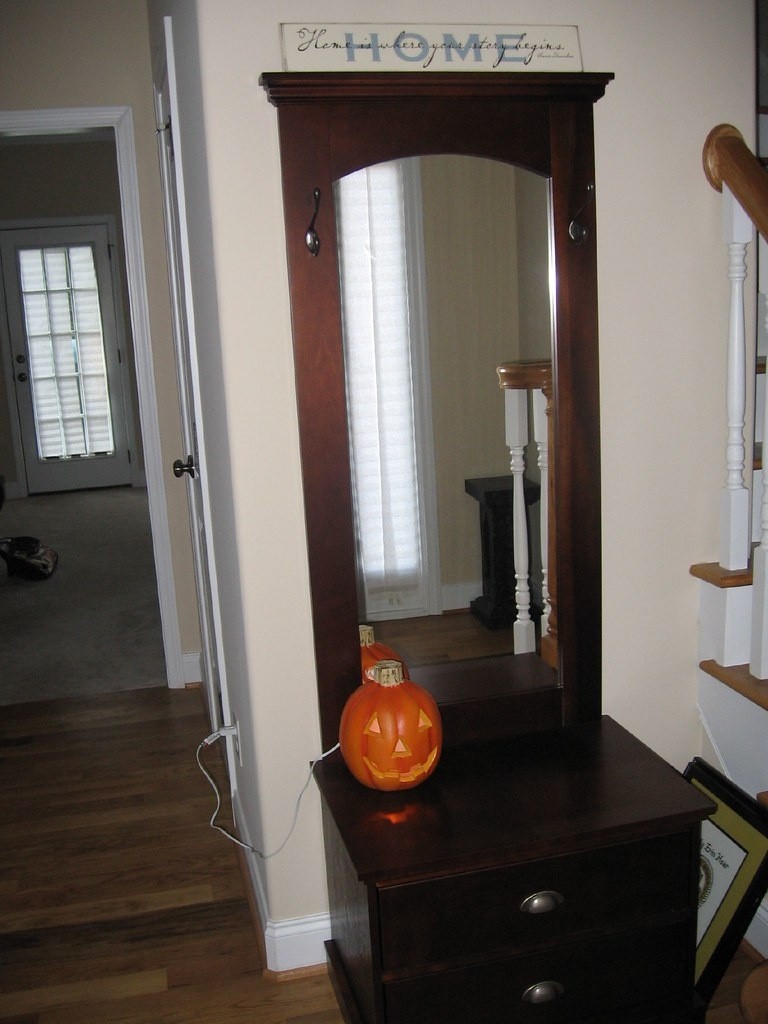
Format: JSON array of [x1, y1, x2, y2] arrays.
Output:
[[331, 153, 557, 707]]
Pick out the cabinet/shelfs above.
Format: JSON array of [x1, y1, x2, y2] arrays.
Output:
[[310, 714, 717, 1024]]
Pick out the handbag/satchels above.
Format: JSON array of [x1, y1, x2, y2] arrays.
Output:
[[0, 537, 59, 580]]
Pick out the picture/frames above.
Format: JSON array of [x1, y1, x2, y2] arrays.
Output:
[[683, 756, 768, 1023]]
